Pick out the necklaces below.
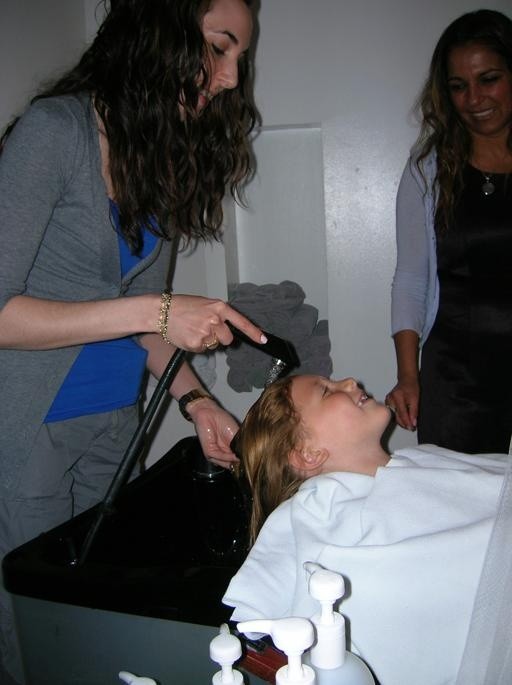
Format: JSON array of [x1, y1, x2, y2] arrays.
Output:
[[473, 152, 508, 197]]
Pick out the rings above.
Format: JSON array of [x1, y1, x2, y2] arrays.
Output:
[[206, 338, 218, 351]]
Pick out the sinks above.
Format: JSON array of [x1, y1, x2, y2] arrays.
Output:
[[2, 434, 244, 685]]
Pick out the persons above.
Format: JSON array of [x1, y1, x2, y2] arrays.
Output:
[[378, 5, 512, 455], [3, 0, 265, 558], [228, 373, 512, 685]]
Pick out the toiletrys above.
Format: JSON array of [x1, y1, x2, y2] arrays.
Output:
[[118, 560, 377, 685]]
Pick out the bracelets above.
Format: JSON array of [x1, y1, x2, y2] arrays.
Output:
[[178, 386, 211, 424], [160, 290, 176, 341]]
